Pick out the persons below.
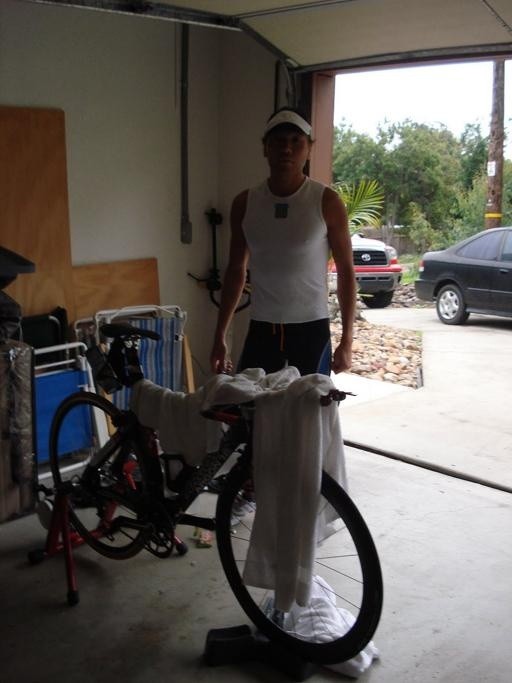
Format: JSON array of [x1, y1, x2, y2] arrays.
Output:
[[207, 105, 357, 528]]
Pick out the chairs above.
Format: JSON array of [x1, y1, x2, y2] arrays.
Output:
[[228, 493, 256, 527]]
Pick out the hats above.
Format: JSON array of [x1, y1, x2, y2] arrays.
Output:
[[414, 226, 512, 326]]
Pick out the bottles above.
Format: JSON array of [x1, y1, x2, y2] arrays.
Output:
[[263, 110, 314, 142]]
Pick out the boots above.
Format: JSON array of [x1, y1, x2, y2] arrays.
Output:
[[327, 233, 403, 308]]
